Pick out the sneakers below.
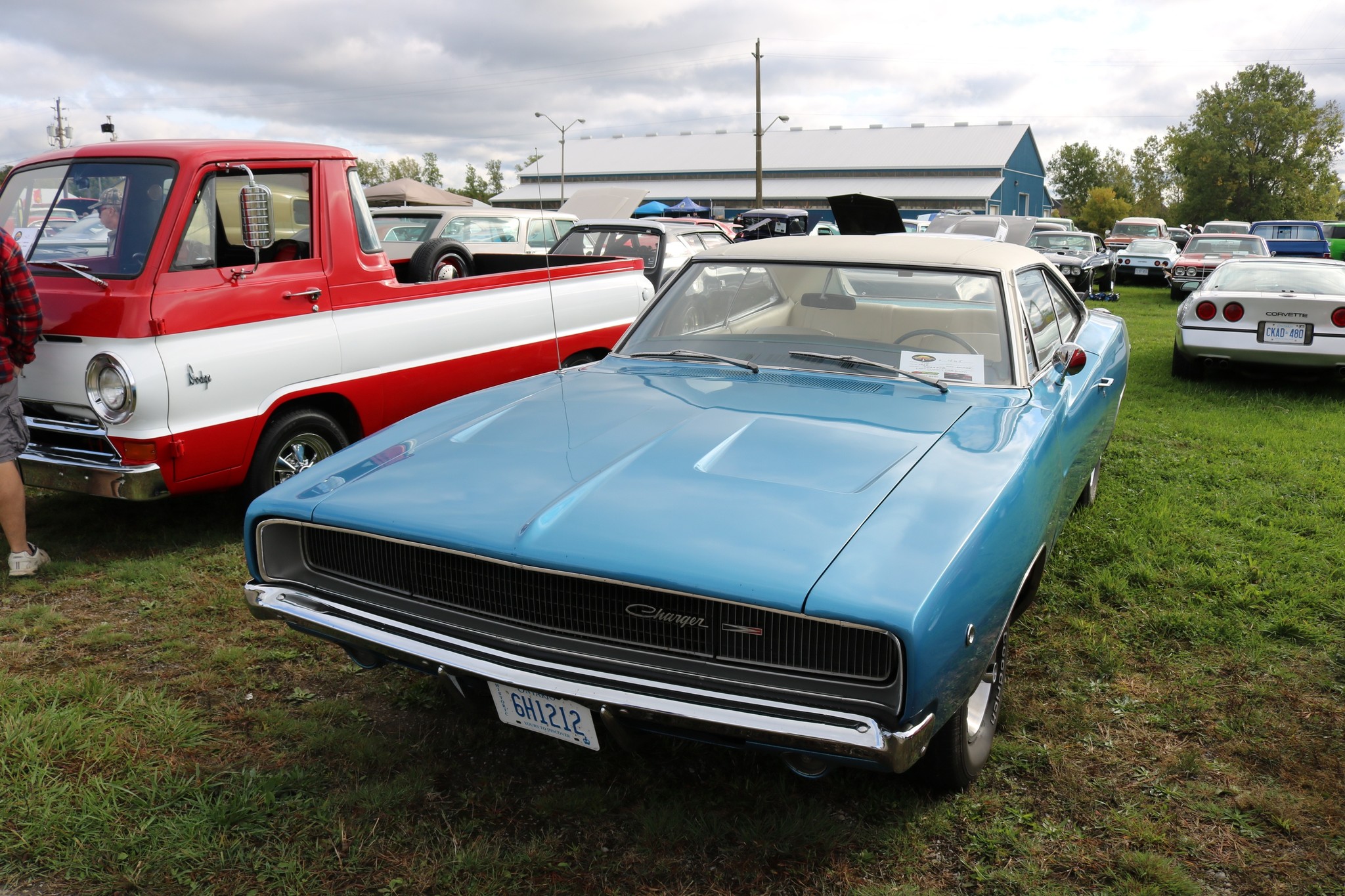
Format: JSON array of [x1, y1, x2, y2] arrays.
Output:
[[8, 541, 52, 576]]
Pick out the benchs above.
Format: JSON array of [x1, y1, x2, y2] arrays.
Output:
[[789, 302, 998, 359]]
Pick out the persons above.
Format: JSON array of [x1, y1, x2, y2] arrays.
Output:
[[0, 228, 52, 576], [686, 212, 744, 234], [1221, 219, 1232, 233], [89, 187, 124, 259], [1183, 224, 1201, 238]]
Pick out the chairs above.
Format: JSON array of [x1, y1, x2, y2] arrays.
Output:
[[919, 333, 1001, 363], [1195, 243, 1213, 253], [745, 326, 835, 337], [607, 245, 632, 257], [1238, 244, 1254, 253]]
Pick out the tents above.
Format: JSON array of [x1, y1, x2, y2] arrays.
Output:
[[364, 177, 474, 208], [634, 197, 711, 219]]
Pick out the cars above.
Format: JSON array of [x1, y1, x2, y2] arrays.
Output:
[[0, 174, 1345, 354], [1170, 257, 1345, 390], [1168, 233, 1278, 300], [1115, 238, 1182, 286], [1023, 230, 1120, 297], [240, 226, 1134, 794]]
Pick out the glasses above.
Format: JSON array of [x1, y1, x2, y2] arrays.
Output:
[[97, 207, 108, 215]]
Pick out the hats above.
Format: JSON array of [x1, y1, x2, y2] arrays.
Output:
[[87, 187, 124, 209]]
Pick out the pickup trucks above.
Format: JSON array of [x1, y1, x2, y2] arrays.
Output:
[[0, 134, 654, 522]]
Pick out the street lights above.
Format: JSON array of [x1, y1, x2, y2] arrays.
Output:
[[755, 115, 789, 209], [535, 111, 586, 207]]
